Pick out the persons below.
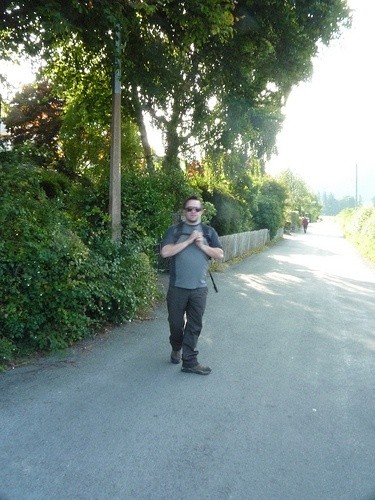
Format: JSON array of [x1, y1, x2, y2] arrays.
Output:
[[160, 196, 225, 375], [301, 215, 310, 234]]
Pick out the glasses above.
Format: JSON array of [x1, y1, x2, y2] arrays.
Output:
[[184, 206, 202, 212]]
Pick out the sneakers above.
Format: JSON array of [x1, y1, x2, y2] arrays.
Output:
[[181, 363, 211, 375], [171, 348, 181, 364]]
